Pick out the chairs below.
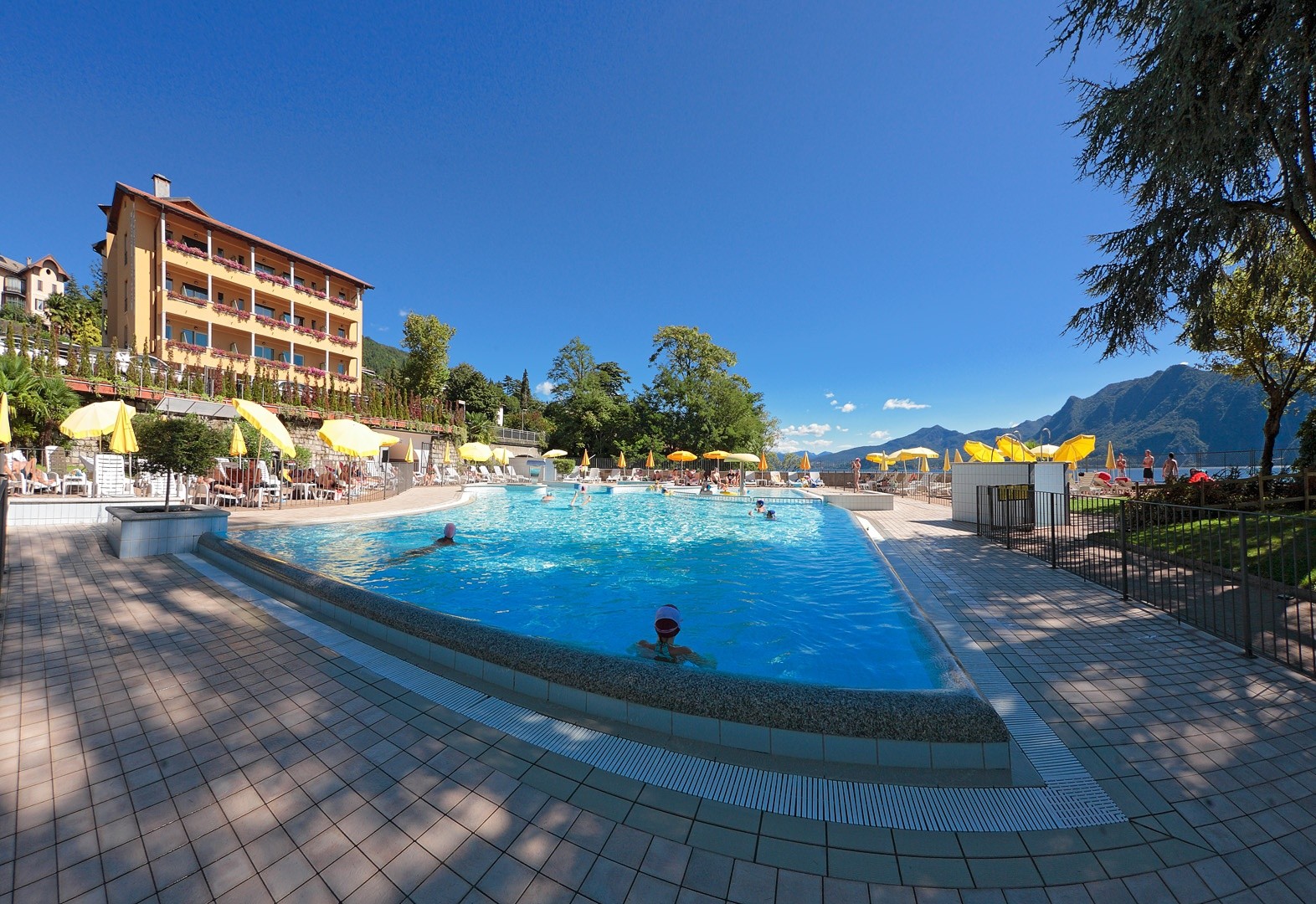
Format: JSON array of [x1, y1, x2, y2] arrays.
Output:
[[583, 468, 601, 484], [606, 468, 622, 484], [728, 469, 740, 487], [434, 464, 530, 483], [690, 472, 700, 487], [746, 471, 825, 488], [79, 454, 136, 497], [654, 470, 663, 479], [710, 471, 721, 487], [629, 468, 643, 482], [190, 459, 398, 509], [564, 465, 582, 483], [864, 472, 952, 499], [45, 445, 87, 495], [1071, 472, 1134, 495], [4, 450, 59, 495]]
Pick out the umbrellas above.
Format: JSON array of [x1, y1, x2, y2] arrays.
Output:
[[229, 398, 297, 471], [646, 450, 811, 477], [582, 448, 590, 470], [59, 399, 140, 454], [444, 440, 514, 475], [542, 449, 568, 458], [405, 437, 414, 463], [0, 392, 12, 448], [618, 451, 627, 476], [318, 419, 401, 460], [866, 433, 1117, 482]]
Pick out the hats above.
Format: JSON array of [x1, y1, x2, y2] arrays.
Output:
[[654, 605, 682, 637], [444, 522, 455, 538], [757, 499, 764, 506], [767, 509, 774, 514]]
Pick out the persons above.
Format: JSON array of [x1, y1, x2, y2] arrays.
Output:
[[424, 462, 484, 485], [197, 459, 371, 501], [748, 500, 778, 521], [4, 453, 57, 496], [626, 604, 718, 670], [541, 490, 593, 507], [850, 457, 917, 492], [1116, 449, 1180, 485], [631, 467, 748, 496]]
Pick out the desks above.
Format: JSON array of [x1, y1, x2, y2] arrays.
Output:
[[61, 474, 87, 498], [757, 480, 767, 487], [721, 478, 727, 480], [675, 479, 685, 486]]
